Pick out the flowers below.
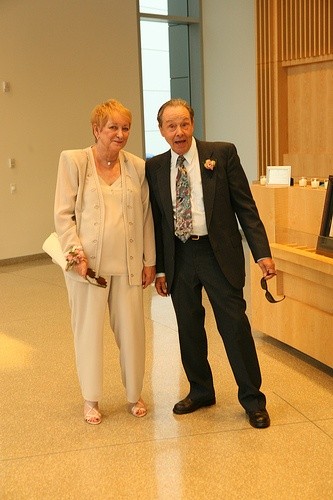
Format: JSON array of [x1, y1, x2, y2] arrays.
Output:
[[204, 159, 217, 171], [64, 246, 88, 278]]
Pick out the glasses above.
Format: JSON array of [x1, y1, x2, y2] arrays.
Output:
[[261, 273, 285, 303], [84, 268, 108, 288]]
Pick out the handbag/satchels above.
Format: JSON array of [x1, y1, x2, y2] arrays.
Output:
[[42, 232, 68, 272]]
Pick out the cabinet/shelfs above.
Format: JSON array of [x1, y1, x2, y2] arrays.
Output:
[[250, 178, 333, 369]]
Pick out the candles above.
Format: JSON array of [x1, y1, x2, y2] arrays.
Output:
[[311, 178, 319, 189], [260, 176, 265, 186], [299, 177, 307, 187], [324, 179, 328, 190]]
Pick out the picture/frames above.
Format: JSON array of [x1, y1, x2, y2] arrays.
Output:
[[265, 166, 292, 187]]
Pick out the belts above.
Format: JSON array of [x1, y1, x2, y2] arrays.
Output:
[[188, 233, 209, 241]]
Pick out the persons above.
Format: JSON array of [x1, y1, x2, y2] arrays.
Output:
[[146, 99, 286, 429], [41, 98, 157, 425]]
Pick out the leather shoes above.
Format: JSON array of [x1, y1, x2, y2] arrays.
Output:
[[173, 393, 216, 414], [246, 406, 271, 428]]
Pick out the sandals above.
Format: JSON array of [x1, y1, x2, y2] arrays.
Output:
[[129, 397, 148, 417], [83, 399, 103, 424]]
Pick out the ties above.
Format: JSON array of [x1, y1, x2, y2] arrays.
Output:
[[174, 155, 194, 243]]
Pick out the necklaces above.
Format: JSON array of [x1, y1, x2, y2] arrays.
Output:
[[104, 157, 118, 166]]
